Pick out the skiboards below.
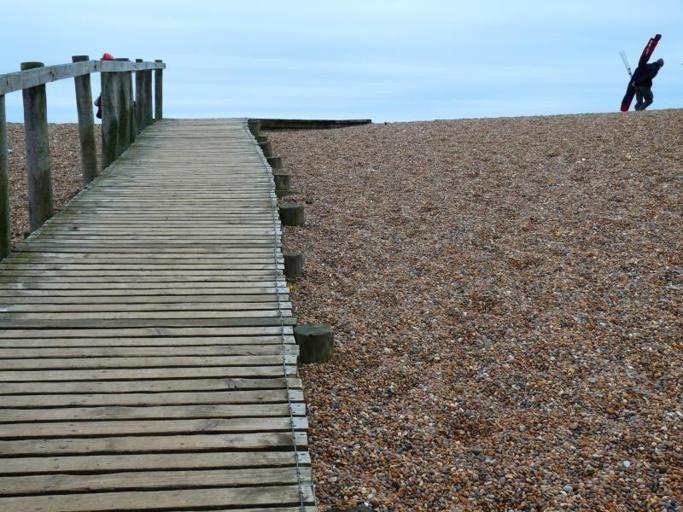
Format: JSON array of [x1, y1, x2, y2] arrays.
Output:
[[620, 32, 662, 111]]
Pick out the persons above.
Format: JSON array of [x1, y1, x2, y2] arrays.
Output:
[[634, 58, 664, 111]]
